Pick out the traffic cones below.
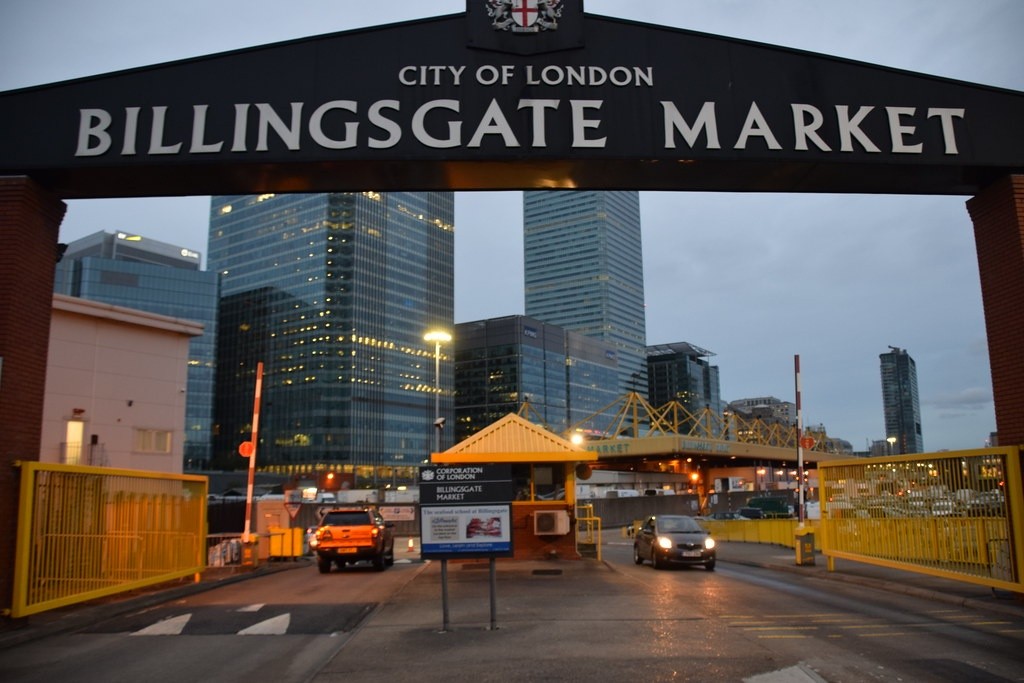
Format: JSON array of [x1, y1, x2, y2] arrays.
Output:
[[408, 536, 415, 553]]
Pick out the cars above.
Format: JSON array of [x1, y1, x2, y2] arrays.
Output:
[[733, 507, 767, 521], [632, 515, 716, 571], [910, 490, 1004, 518]]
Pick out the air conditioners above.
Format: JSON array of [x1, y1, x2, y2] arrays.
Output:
[[534, 509, 570, 536]]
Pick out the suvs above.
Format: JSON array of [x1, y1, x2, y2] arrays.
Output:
[[314, 508, 395, 571]]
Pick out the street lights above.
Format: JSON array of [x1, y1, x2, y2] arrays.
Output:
[[887, 437, 896, 455], [423, 329, 454, 449]]
